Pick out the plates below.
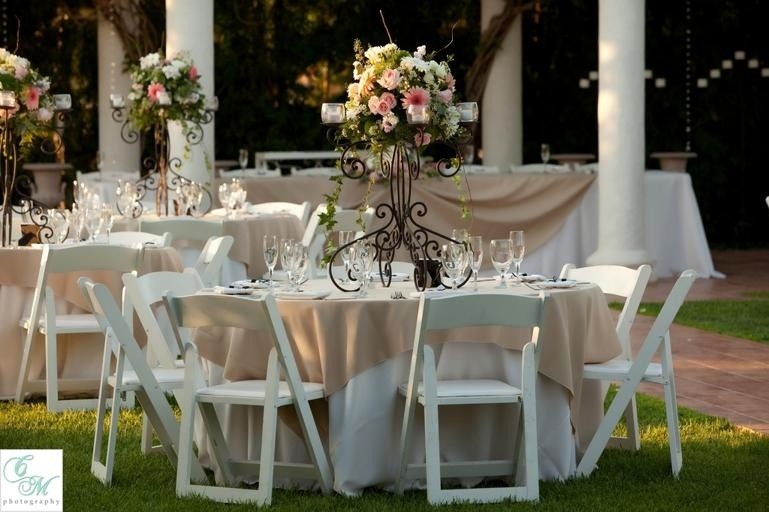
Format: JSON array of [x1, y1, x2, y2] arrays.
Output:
[[232, 278, 274, 289], [369, 272, 408, 282], [277, 289, 330, 301], [409, 291, 440, 299]]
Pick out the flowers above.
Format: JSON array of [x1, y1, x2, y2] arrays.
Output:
[[107, 43, 223, 172], [315, 40, 475, 267], [0, 45, 77, 165]]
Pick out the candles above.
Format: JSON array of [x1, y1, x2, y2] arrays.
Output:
[[0, 95, 12, 106], [412, 112, 426, 122], [55, 100, 69, 108], [159, 94, 169, 103], [207, 101, 217, 109], [111, 96, 122, 106], [327, 111, 341, 122], [461, 110, 472, 119]]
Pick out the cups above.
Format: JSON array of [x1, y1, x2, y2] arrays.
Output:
[[73, 181, 89, 206]]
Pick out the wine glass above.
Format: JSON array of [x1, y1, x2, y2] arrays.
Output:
[[465, 144, 475, 174], [510, 230, 526, 287], [260, 234, 310, 292], [218, 178, 248, 221], [338, 230, 372, 299], [239, 149, 249, 179], [489, 238, 514, 289], [541, 143, 550, 173], [19, 198, 115, 246], [441, 228, 483, 295], [119, 181, 136, 221], [174, 178, 202, 221]]
